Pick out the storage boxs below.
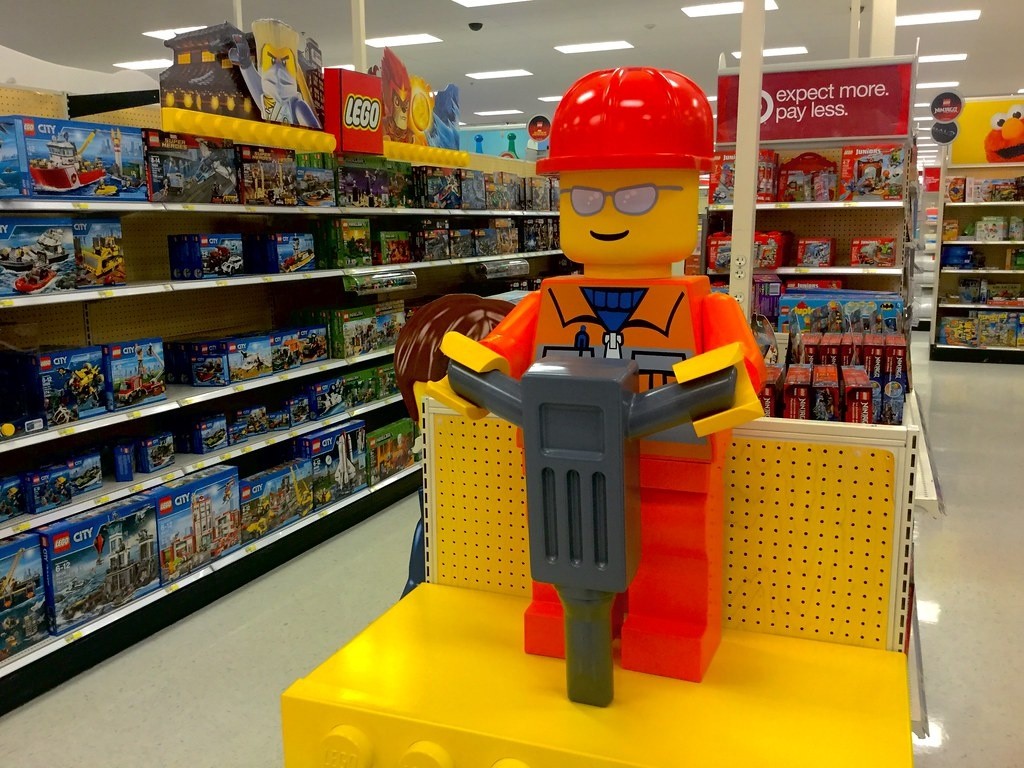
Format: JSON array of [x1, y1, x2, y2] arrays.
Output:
[[708, 142, 907, 425], [0, 116, 582, 660], [939, 177, 1024, 348]]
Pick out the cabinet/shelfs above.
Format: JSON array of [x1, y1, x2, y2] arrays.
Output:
[[929, 96, 1024, 365], [911, 93, 940, 332], [700, 37, 939, 517], [0, 88, 583, 716]]
